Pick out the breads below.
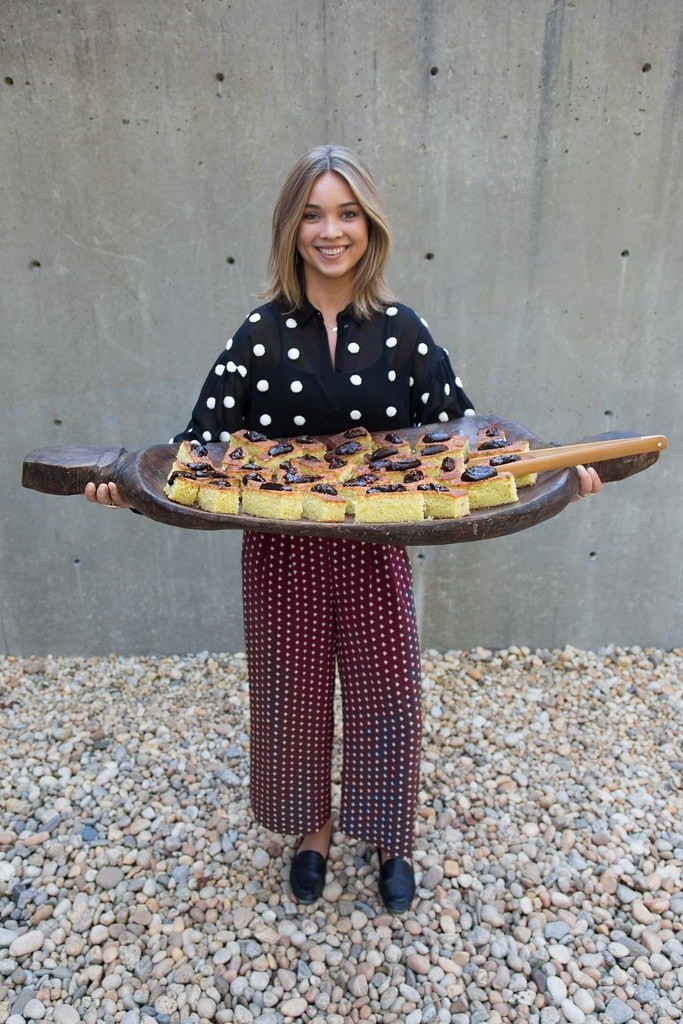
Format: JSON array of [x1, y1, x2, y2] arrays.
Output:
[[162, 425, 537, 522]]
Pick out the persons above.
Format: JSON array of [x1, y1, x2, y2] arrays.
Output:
[[85, 145, 602, 916]]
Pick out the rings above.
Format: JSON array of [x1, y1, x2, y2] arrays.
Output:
[[102, 502, 121, 509], [592, 491, 598, 496], [578, 490, 588, 499]]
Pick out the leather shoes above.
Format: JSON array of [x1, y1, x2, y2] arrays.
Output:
[[289, 827, 331, 904], [377, 846, 415, 914]]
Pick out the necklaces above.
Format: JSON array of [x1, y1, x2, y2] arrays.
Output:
[[325, 325, 338, 332]]
